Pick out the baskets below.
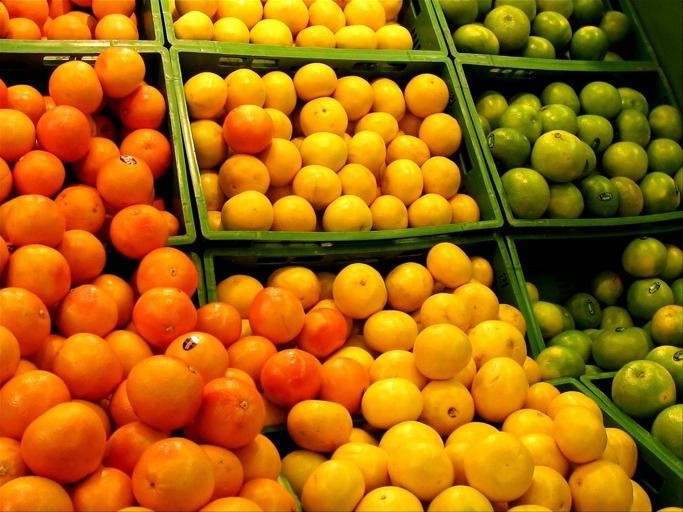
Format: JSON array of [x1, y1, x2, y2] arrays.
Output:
[[0, 0, 683, 512]]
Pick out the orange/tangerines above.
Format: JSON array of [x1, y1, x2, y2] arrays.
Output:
[[0, 0, 683, 512]]
